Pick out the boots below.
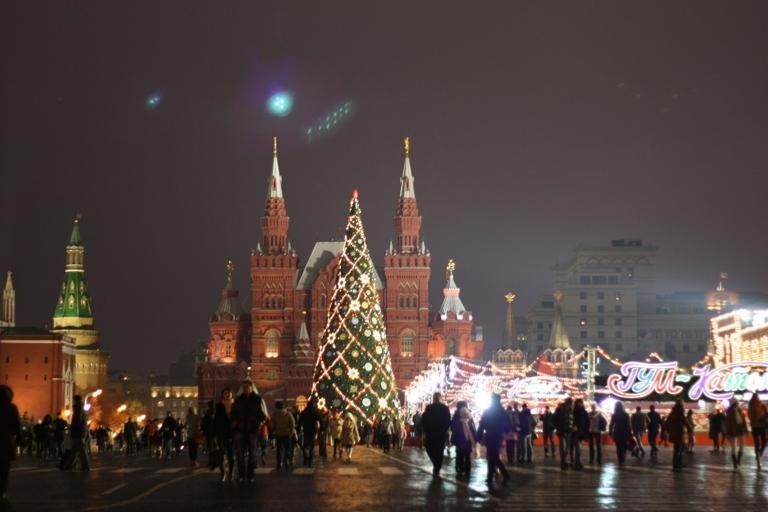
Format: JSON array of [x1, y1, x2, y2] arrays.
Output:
[[218, 455, 226, 482], [226, 456, 235, 482]]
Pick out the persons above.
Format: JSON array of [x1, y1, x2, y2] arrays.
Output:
[[1, 381, 361, 512], [362, 391, 768, 495]]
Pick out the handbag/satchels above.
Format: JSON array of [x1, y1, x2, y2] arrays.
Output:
[[207, 448, 220, 471]]
